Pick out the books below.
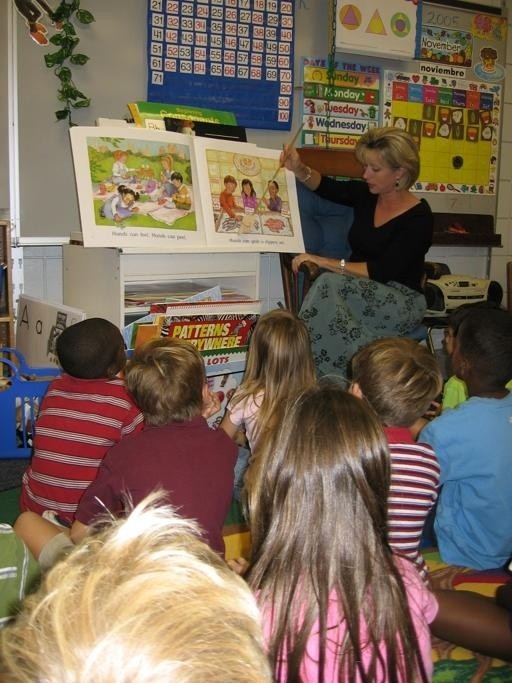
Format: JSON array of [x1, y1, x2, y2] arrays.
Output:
[[68, 99, 308, 433]]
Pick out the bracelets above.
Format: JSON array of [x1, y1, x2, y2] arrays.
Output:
[[296, 164, 312, 184]]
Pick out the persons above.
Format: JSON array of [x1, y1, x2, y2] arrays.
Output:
[[280, 128, 434, 378], [0, 299, 511, 681]]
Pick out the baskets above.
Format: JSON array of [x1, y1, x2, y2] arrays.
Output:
[[0, 348, 63, 458]]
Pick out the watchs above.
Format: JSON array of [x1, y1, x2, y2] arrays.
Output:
[[340, 255, 346, 276]]
